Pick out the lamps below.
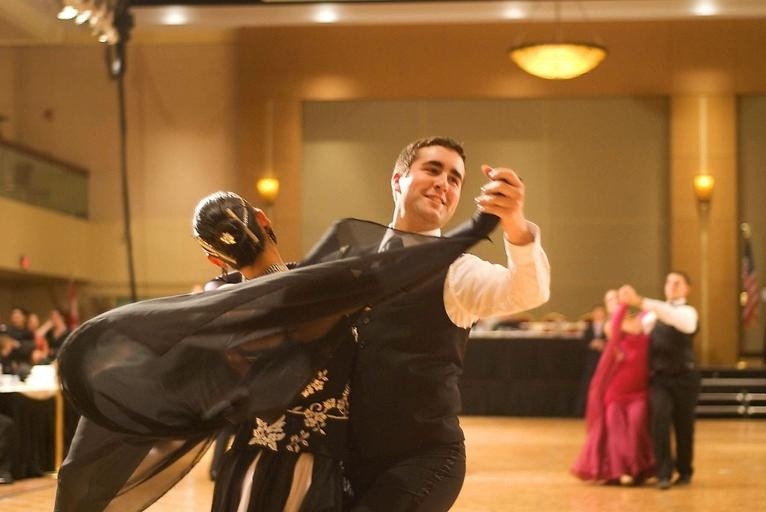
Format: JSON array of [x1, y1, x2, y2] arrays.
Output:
[[693, 174, 715, 222], [506, 1, 608, 82], [42, 0, 121, 45]]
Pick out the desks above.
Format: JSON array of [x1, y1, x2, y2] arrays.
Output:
[[0, 390, 81, 484], [456, 335, 605, 417]]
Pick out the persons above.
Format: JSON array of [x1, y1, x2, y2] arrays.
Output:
[[214, 133, 551, 512], [570, 301, 607, 418], [567, 286, 660, 488], [618, 270, 701, 488], [0, 292, 119, 385], [51, 172, 516, 511]]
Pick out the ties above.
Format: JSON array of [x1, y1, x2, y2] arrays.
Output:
[[382, 236, 404, 252]]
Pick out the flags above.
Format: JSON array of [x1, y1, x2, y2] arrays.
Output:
[[737, 224, 761, 331]]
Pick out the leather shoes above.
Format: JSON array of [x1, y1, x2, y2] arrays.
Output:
[[673, 468, 693, 486], [656, 477, 673, 489]]
